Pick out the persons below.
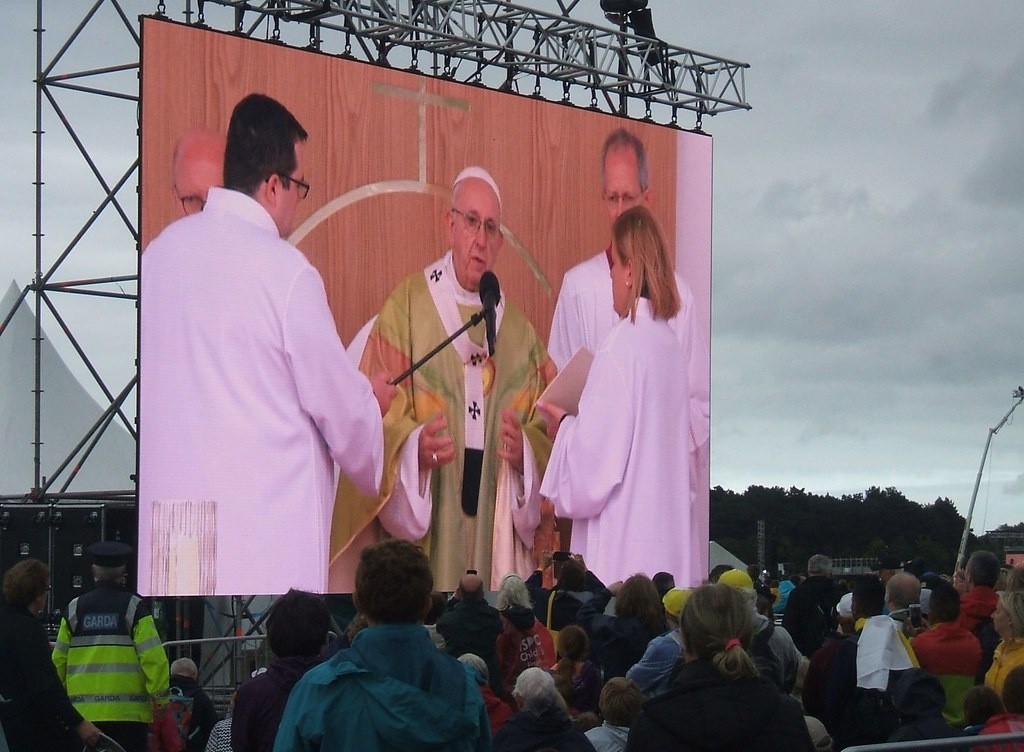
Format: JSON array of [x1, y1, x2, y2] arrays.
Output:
[[718, 568, 801, 700], [574, 573, 668, 687], [548, 130, 710, 522], [329, 164, 557, 593], [168, 658, 218, 752], [910, 585, 985, 724], [0, 559, 102, 752], [584, 676, 640, 752], [424, 551, 1024, 752], [273, 540, 492, 752], [532, 206, 695, 589], [51, 541, 170, 752], [624, 583, 816, 752], [230, 587, 330, 752], [140, 92, 385, 593], [492, 667, 597, 752], [435, 573, 503, 671], [206, 691, 237, 752], [173, 130, 225, 218]]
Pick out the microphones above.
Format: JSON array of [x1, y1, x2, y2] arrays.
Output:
[[479, 270, 501, 357]]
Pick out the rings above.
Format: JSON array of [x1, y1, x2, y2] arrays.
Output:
[[432, 453, 438, 463]]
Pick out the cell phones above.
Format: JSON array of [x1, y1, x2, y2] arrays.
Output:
[[553, 551, 570, 561], [467, 570, 477, 575], [909, 604, 922, 628]]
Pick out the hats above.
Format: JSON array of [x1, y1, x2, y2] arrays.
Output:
[[804, 715, 832, 750], [266, 588, 331, 659], [170, 658, 198, 681], [663, 588, 693, 618], [836, 592, 853, 617], [87, 541, 131, 567], [500, 605, 535, 630], [718, 569, 754, 590], [920, 571, 945, 588], [252, 667, 266, 677], [452, 167, 503, 213]]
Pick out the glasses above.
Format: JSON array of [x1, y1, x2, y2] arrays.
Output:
[[451, 209, 499, 237], [602, 189, 649, 205], [953, 581, 958, 584], [173, 184, 205, 216], [44, 584, 51, 590], [265, 170, 310, 199]]
